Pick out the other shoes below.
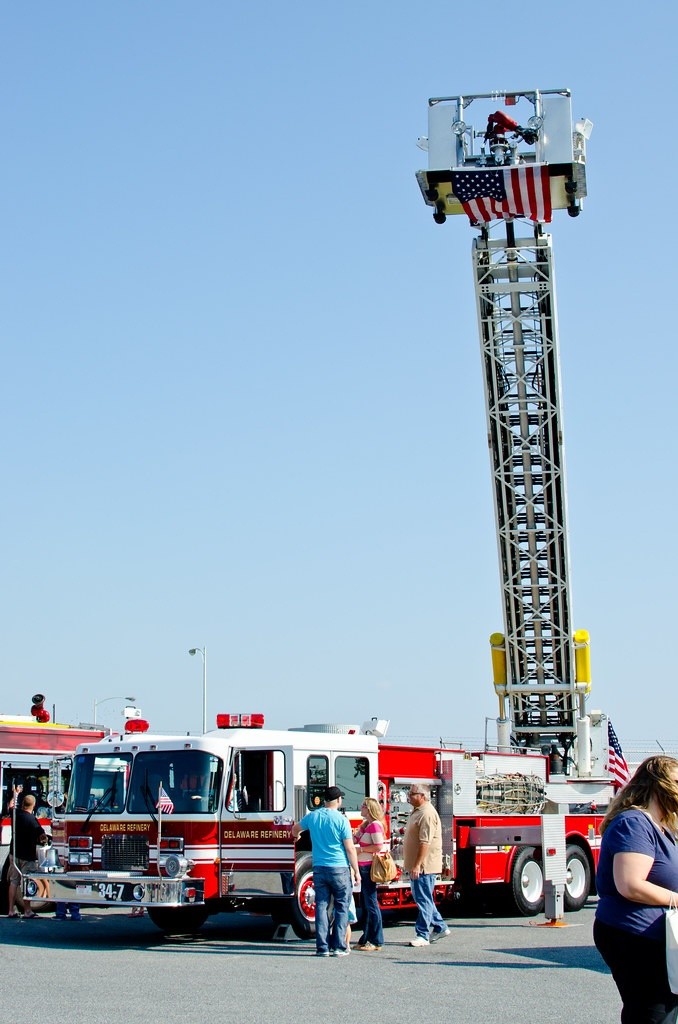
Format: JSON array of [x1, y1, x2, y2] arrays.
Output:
[[346, 895, 357, 924], [350, 941, 381, 950], [50, 915, 66, 920], [71, 914, 82, 921]]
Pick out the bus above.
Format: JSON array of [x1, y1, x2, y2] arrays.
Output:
[[0, 715, 108, 911]]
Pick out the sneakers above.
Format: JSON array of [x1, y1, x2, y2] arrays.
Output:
[[429, 928, 451, 943], [408, 936, 430, 947], [332, 948, 349, 957], [316, 949, 330, 957]]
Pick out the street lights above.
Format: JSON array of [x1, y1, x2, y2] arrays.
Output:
[[189, 648, 206, 735], [94, 697, 136, 729]]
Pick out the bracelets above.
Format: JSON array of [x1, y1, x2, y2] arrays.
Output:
[[361, 848, 362, 852]]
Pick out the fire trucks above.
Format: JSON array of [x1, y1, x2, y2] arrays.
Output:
[[8, 88, 616, 942]]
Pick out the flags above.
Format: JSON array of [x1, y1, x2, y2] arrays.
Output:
[[156, 788, 174, 814], [451, 165, 552, 223], [607, 722, 631, 788]]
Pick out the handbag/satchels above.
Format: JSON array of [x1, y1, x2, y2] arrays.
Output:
[[371, 850, 397, 883], [665, 890, 678, 994]]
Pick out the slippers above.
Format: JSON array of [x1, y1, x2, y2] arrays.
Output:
[[22, 911, 42, 919], [7, 912, 20, 918]]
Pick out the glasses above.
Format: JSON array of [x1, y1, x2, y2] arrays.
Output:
[[408, 790, 421, 796], [361, 804, 367, 810]]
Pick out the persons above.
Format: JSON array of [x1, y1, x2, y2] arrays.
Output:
[[593, 756, 678, 1024], [403, 783, 451, 947], [353, 797, 388, 950], [51, 902, 82, 921], [292, 787, 360, 957], [7, 784, 45, 919], [128, 907, 144, 918]]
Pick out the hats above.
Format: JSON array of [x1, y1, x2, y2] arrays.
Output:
[[324, 785, 345, 802]]
